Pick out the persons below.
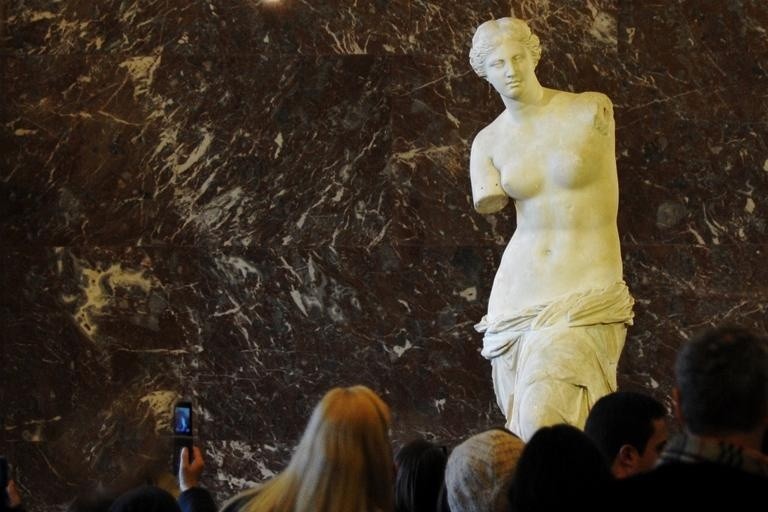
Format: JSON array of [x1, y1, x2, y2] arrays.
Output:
[[594, 323, 767, 511], [1, 385, 669, 511], [466, 16, 638, 444]]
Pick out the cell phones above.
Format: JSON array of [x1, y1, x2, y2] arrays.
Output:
[[173, 403, 193, 471]]
